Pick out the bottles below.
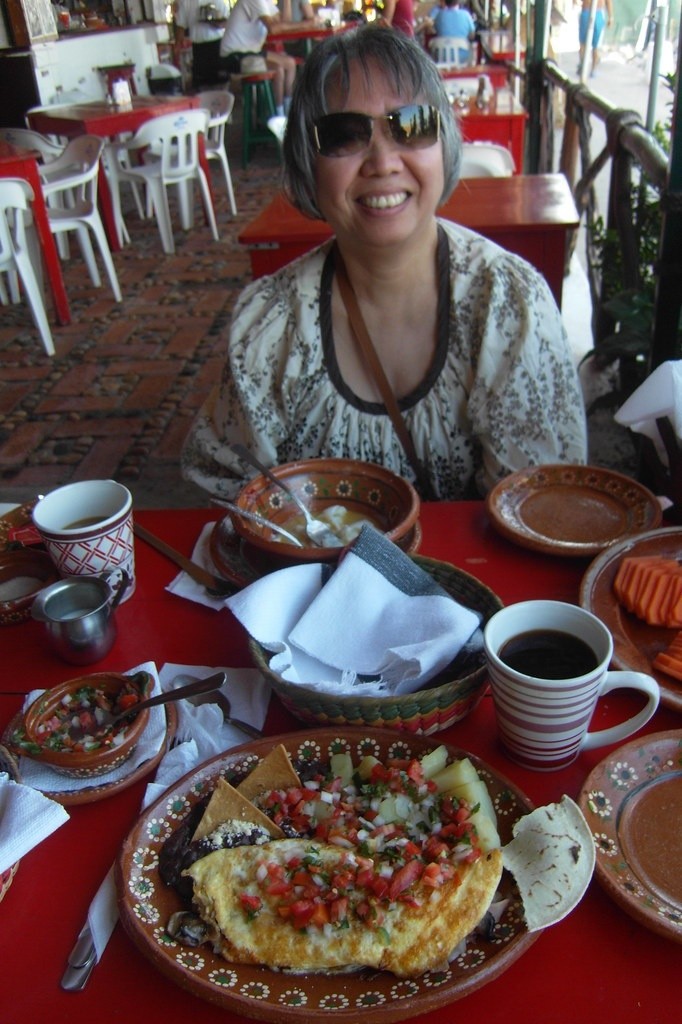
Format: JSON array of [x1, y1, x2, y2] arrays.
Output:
[[476, 75, 490, 109], [106, 77, 131, 107]]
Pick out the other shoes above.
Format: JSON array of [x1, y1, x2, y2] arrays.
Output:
[[225, 113, 233, 127], [589, 71, 595, 78], [576, 62, 582, 75]]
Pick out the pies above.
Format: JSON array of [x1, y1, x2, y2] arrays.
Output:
[[183, 837, 502, 979]]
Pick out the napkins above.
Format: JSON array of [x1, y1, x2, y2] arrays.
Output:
[[615, 357, 682, 477], [163, 522, 238, 612], [76, 657, 271, 964]]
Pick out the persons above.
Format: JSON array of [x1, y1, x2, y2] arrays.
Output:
[[217, 0, 321, 120], [572, 0, 613, 79], [338, 12, 365, 36], [174, 0, 239, 93], [380, 0, 487, 67], [172, 24, 587, 507]]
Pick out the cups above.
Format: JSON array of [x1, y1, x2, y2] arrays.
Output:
[[483, 599, 660, 773], [32, 479, 135, 605]]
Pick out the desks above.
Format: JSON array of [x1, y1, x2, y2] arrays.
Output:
[[28, 94, 216, 249], [455, 83, 531, 174], [237, 172, 581, 314], [0, 140, 70, 326], [437, 63, 508, 92]]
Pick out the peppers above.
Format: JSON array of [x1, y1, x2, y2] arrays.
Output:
[[238, 758, 481, 923]]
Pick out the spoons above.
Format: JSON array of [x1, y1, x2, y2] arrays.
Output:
[[133, 519, 240, 597], [171, 674, 269, 738], [53, 671, 226, 745], [230, 443, 336, 547]]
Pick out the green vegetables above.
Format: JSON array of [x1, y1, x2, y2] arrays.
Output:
[[12, 678, 135, 754]]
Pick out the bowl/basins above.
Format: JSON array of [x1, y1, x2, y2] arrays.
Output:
[[229, 459, 420, 569], [1, 539, 57, 627], [11, 672, 155, 778]]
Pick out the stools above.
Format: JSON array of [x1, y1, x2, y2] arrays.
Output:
[[242, 69, 286, 169]]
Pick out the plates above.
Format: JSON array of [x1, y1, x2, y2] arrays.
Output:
[[119, 726, 547, 1022], [0, 685, 176, 807], [486, 463, 662, 558], [211, 518, 422, 585], [579, 525, 681, 715], [577, 726, 682, 946]]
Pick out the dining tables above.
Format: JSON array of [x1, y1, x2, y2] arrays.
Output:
[[0, 495, 682, 1024]]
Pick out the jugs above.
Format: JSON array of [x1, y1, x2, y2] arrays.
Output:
[[32, 566, 130, 667]]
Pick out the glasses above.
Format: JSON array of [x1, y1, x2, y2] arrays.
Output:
[[309, 104, 442, 158]]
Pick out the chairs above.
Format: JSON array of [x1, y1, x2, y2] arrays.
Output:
[[0, 34, 516, 357]]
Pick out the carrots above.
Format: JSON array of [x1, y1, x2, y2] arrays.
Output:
[[615, 554, 682, 681]]
